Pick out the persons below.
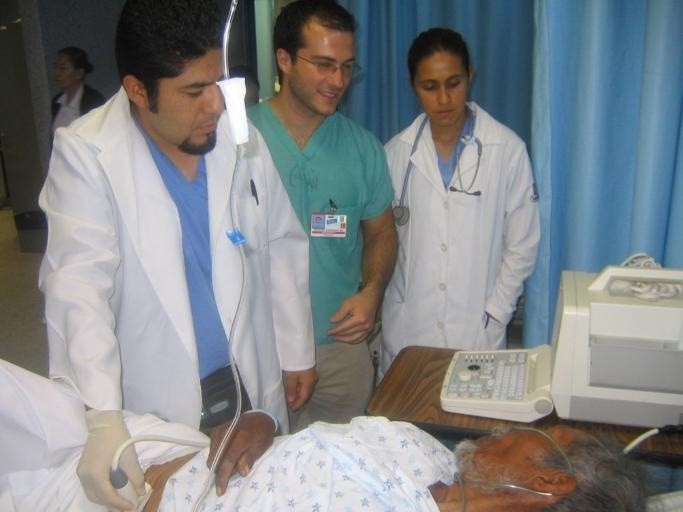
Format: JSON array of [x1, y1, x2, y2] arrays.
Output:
[[76, 408, 664, 512], [30, 0, 318, 511], [360, 27, 542, 388], [246, 0, 399, 432], [49, 48, 105, 147]]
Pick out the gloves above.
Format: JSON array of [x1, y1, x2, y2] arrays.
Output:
[[77, 409, 145, 512]]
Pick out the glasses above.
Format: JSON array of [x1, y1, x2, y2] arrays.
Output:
[[296, 55, 361, 77]]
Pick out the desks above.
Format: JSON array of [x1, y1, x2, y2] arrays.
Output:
[[365, 344, 683, 463]]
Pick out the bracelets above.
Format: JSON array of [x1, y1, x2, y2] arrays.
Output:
[[240, 409, 278, 433]]
